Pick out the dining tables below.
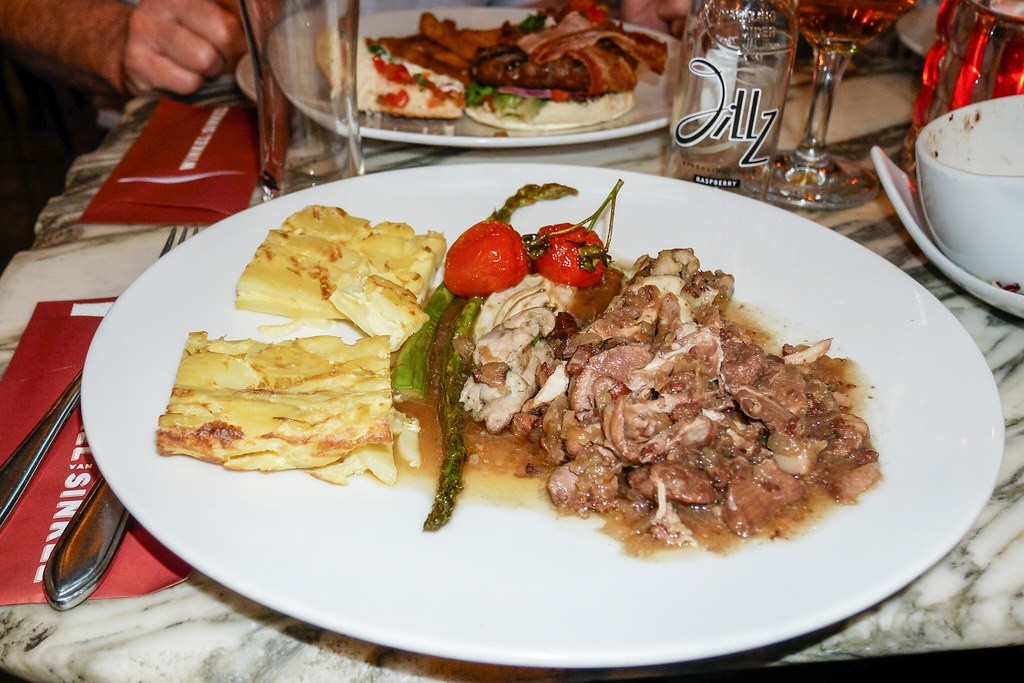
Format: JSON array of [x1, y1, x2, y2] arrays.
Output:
[[0, 17, 1024, 683]]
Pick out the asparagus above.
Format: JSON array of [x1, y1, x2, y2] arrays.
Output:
[[392, 182, 579, 531]]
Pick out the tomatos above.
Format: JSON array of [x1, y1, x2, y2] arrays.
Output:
[[532, 223, 607, 290], [443, 219, 530, 299]]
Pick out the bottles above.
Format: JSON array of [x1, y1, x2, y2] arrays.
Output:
[[663, 0, 798, 203]]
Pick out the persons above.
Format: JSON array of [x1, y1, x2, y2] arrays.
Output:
[[0, 0, 692, 199]]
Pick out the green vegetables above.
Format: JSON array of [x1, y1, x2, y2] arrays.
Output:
[[369, 14, 590, 119]]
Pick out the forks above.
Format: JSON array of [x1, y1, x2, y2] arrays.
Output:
[[0, 227, 200, 529]]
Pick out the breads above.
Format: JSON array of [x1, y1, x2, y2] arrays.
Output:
[[467, 90, 636, 130], [315, 29, 466, 119]]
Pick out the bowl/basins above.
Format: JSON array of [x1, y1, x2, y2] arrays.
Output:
[[914, 94, 1023, 295]]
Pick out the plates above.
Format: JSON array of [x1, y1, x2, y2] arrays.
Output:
[[870, 143, 1023, 319], [897, 6, 941, 55], [80, 162, 1005, 672], [236, 10, 680, 146]]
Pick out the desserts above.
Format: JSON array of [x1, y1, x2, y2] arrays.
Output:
[[154, 331, 420, 486], [234, 205, 447, 348]]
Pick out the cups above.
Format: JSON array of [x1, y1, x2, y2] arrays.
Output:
[[241, 0, 364, 201]]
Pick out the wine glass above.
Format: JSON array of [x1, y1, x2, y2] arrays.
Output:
[[735, 0, 919, 207]]
